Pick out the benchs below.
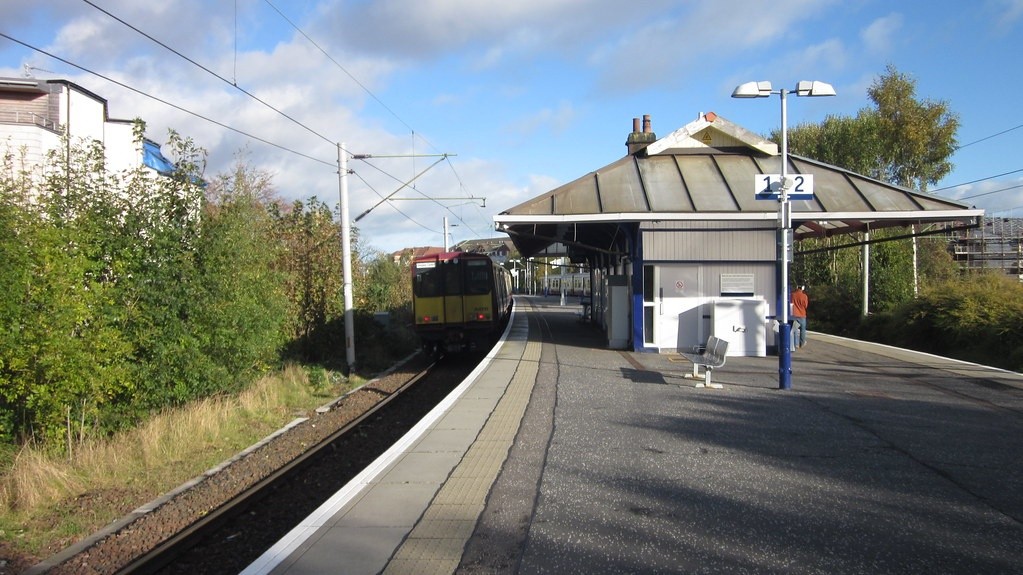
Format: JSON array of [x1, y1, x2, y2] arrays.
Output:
[[680, 335, 730, 388]]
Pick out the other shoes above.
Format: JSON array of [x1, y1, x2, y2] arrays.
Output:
[[795, 345, 799, 349], [801, 341, 807, 348]]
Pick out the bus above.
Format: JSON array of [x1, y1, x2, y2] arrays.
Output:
[[411, 252, 513, 345]]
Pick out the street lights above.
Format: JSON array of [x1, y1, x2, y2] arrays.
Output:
[[509, 260, 520, 294], [732, 80, 837, 389]]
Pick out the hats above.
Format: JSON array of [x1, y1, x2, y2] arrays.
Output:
[[796, 284, 805, 290]]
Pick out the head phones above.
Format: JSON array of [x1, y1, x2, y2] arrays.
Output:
[[797, 285, 805, 290]]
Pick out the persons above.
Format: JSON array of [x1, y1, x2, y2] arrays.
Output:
[[791, 283, 808, 349]]
